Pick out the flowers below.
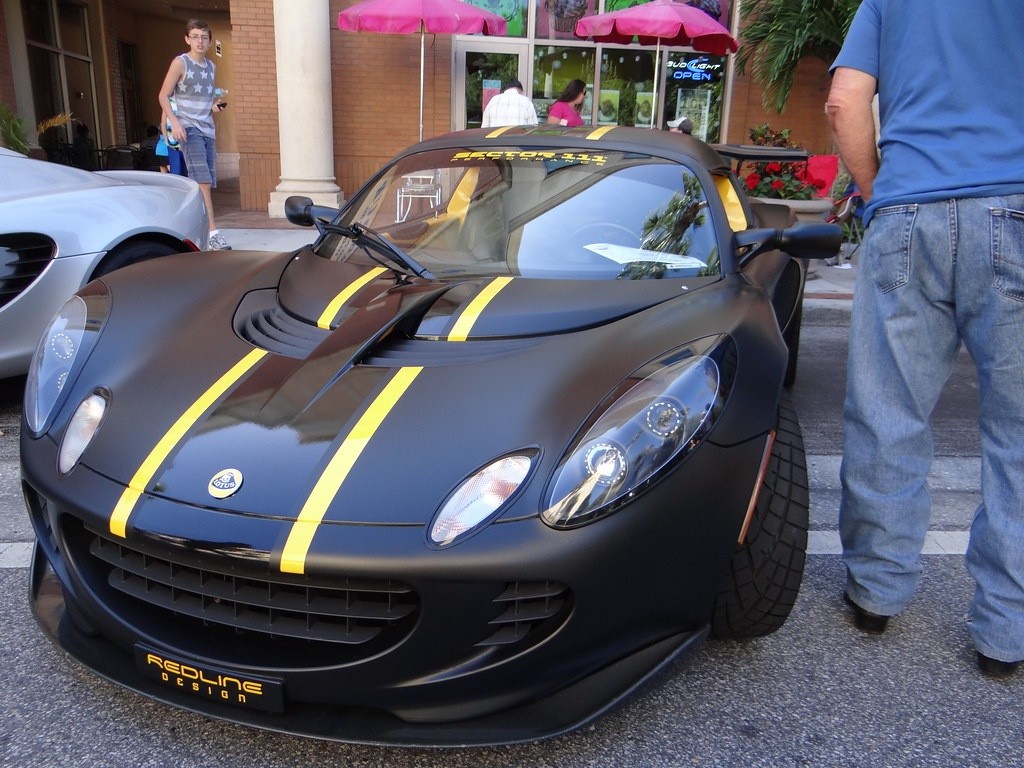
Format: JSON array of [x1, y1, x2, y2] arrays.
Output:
[[733, 127, 825, 200]]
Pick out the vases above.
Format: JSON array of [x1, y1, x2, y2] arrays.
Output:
[[749, 199, 834, 269]]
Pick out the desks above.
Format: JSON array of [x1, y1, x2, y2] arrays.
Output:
[[395, 169, 441, 223]]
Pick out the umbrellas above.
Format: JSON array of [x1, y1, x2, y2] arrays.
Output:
[[337, 0, 506, 212], [573, 0, 738, 129]]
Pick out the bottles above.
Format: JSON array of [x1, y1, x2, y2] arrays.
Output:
[[213, 88, 228, 97]]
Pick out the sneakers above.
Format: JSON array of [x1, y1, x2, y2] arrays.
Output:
[[207, 233, 231, 251]]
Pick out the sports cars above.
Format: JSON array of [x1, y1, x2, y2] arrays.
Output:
[[20, 124, 845, 747], [0, 144, 212, 382]]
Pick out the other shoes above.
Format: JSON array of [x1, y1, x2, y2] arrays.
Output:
[[844, 590, 889, 636], [977, 652, 1024, 678]]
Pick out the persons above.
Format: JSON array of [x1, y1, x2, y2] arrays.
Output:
[[636, 99, 656, 124], [827, 0, 1024, 677], [481, 79, 538, 128], [155, 19, 232, 251], [597, 99, 617, 122], [690, 99, 698, 108], [547, 79, 586, 127], [667, 117, 693, 135]]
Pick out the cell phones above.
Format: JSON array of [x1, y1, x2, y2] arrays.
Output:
[[216, 102, 227, 110]]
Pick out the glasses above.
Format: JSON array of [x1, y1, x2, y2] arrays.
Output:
[[188, 35, 211, 41], [580, 90, 586, 96]]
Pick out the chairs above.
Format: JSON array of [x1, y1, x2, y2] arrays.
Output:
[[103, 143, 160, 171], [746, 155, 868, 270]]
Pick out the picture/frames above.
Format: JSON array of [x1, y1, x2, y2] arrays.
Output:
[[635, 93, 657, 126], [677, 88, 710, 142], [597, 91, 620, 126]]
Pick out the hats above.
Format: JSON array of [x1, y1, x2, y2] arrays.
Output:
[[667, 116, 693, 134]]
